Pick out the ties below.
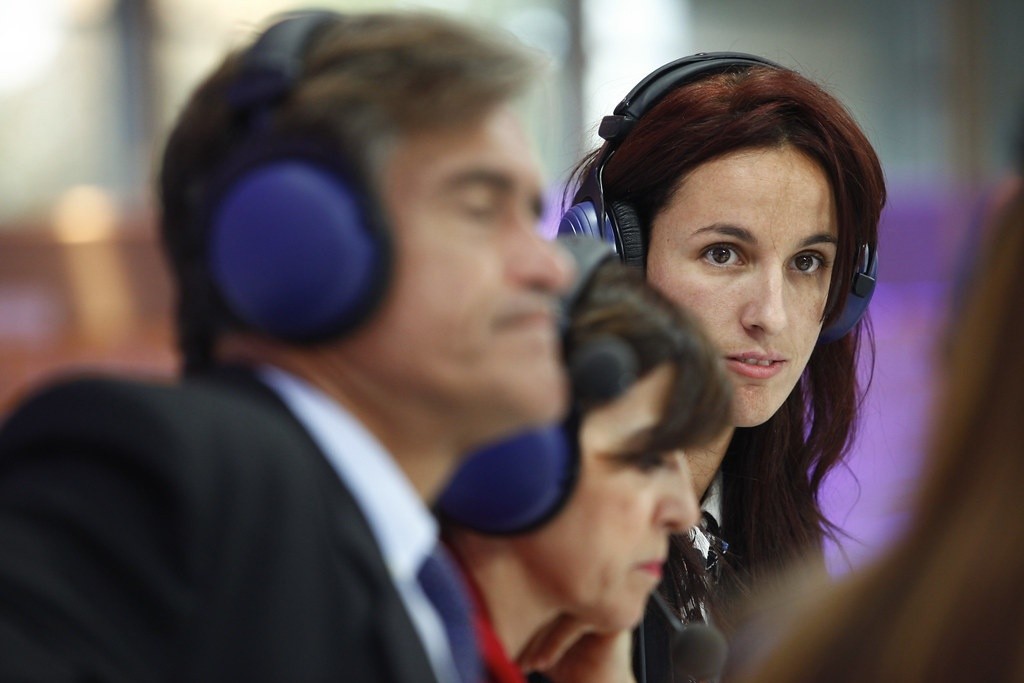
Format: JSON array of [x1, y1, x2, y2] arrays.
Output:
[[420, 540, 488, 683]]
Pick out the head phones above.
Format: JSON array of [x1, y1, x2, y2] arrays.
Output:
[[559, 52, 880, 341], [205, 7, 392, 345], [426, 236, 617, 539]]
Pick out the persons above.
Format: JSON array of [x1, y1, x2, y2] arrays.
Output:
[[737, 171, 1024, 683], [0, 4, 573, 683], [431, 260, 734, 683], [558, 53, 888, 683]]
